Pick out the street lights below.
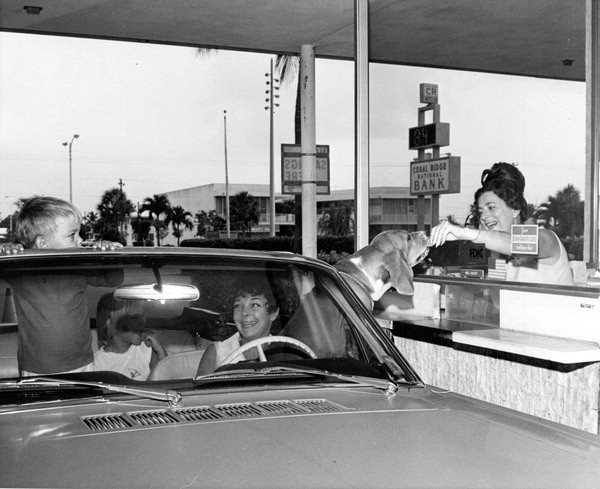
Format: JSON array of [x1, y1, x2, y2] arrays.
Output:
[[61, 133, 80, 205], [224, 110, 230, 238], [264, 58, 281, 235]]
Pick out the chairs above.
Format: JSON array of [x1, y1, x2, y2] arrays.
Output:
[[105, 269, 215, 382]]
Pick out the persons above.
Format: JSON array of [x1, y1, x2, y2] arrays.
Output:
[[94, 291, 168, 382], [0, 196, 124, 377], [194, 274, 282, 380], [430, 162, 574, 286]]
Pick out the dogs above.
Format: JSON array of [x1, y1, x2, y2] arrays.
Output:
[[256, 228, 431, 363]]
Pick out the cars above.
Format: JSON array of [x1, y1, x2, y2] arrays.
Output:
[[1, 246, 600, 489]]
